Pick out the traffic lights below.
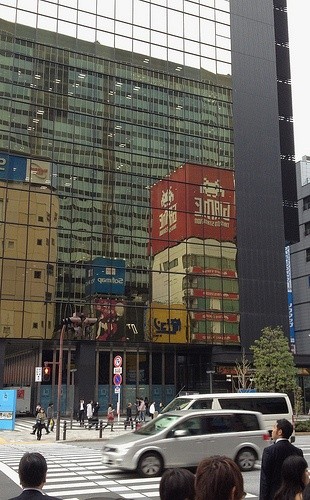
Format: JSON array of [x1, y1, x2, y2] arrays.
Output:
[[86, 318, 98, 330], [62, 316, 81, 324], [43, 367, 51, 382]]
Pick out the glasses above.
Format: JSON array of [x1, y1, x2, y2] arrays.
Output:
[[236, 492, 246, 500]]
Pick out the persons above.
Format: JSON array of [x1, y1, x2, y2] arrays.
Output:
[[160, 419, 310, 500], [30, 402, 55, 435], [78, 397, 156, 432], [8, 452, 63, 500]]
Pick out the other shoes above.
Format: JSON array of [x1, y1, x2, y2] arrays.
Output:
[[37, 438, 41, 440], [30, 433, 35, 435], [47, 431, 49, 434]]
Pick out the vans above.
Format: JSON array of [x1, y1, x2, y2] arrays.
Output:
[[153, 393, 296, 450], [101, 409, 271, 478]]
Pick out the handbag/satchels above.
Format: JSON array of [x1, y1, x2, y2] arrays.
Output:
[[107, 412, 114, 420], [37, 420, 41, 428], [124, 416, 130, 426]]
